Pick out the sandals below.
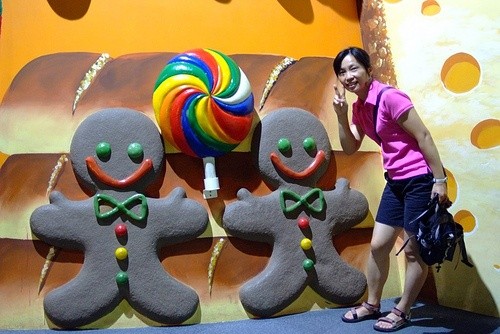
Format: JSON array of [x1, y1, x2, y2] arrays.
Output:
[[341, 301, 382, 323], [373, 307, 412, 332]]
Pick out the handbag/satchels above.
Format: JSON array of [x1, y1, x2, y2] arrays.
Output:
[[395, 193, 474, 273]]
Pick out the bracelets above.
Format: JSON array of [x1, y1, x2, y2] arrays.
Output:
[[433, 177, 448, 184]]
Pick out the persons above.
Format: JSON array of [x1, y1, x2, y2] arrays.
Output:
[[332, 47, 448, 332]]
